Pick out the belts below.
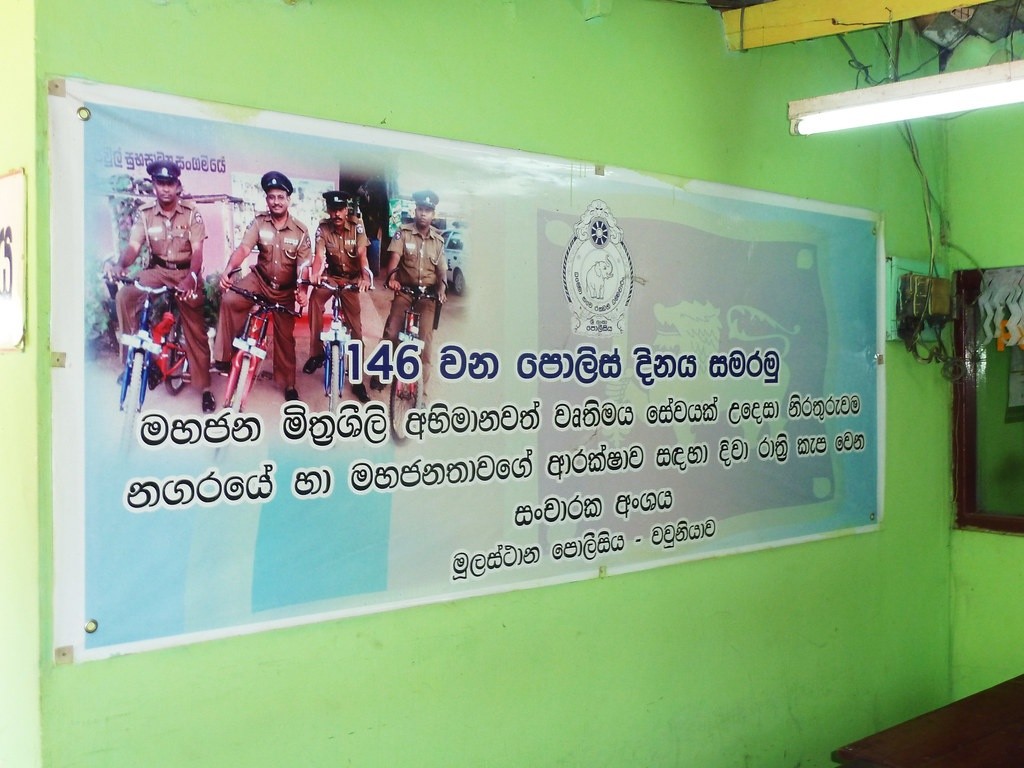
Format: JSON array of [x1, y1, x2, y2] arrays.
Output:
[[152, 254, 189, 269], [410, 286, 432, 293], [256, 264, 292, 290], [333, 271, 359, 278]]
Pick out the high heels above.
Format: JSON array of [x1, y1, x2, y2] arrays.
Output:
[[285, 386, 298, 400]]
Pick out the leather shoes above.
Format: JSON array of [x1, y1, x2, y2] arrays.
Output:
[[370, 375, 387, 391], [118, 370, 131, 386], [352, 383, 370, 404], [215, 361, 230, 373], [202, 390, 216, 413], [303, 353, 327, 374]]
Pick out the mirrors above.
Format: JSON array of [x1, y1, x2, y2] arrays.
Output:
[[952, 266, 1023, 535]]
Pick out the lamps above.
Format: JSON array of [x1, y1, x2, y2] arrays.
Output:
[[787, 59, 1024, 136]]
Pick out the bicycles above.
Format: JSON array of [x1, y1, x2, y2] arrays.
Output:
[[103, 273, 198, 460], [296, 260, 375, 415], [372, 267, 448, 447], [223, 268, 306, 415]]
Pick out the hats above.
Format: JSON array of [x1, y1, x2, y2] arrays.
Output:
[[261, 171, 293, 195], [412, 189, 438, 209], [323, 190, 350, 211], [147, 160, 181, 182]]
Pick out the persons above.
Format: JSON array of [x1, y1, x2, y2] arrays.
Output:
[[302, 190, 372, 403], [211, 171, 312, 401], [369, 190, 448, 409], [99, 161, 215, 415]]
